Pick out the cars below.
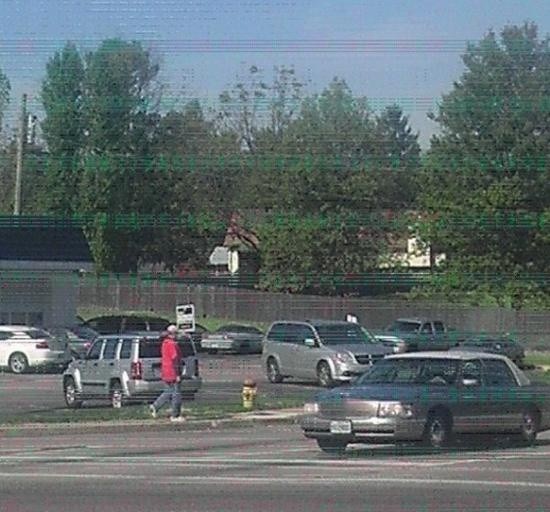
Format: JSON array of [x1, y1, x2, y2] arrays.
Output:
[[61, 331, 201, 408], [0, 324, 64, 408], [262, 317, 550, 452], [200, 323, 264, 354]]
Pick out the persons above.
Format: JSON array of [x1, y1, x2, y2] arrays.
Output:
[[148, 324, 187, 422]]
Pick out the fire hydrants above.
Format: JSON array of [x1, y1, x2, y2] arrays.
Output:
[[240, 376, 258, 409]]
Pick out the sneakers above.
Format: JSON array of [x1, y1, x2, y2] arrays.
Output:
[[170, 415, 185, 424], [148, 403, 158, 419]]
